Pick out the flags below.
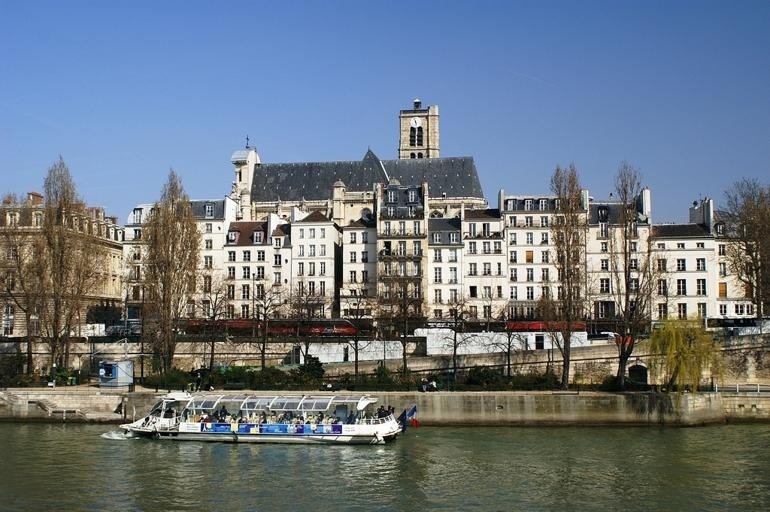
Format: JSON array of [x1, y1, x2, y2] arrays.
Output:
[[397, 405, 418, 432]]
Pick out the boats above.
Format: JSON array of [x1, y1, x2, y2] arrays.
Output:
[[119, 393, 403, 445]]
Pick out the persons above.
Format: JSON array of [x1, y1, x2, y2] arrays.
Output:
[[167, 406, 361, 424], [418, 377, 438, 392], [319, 382, 340, 391], [377, 405, 395, 418]]
